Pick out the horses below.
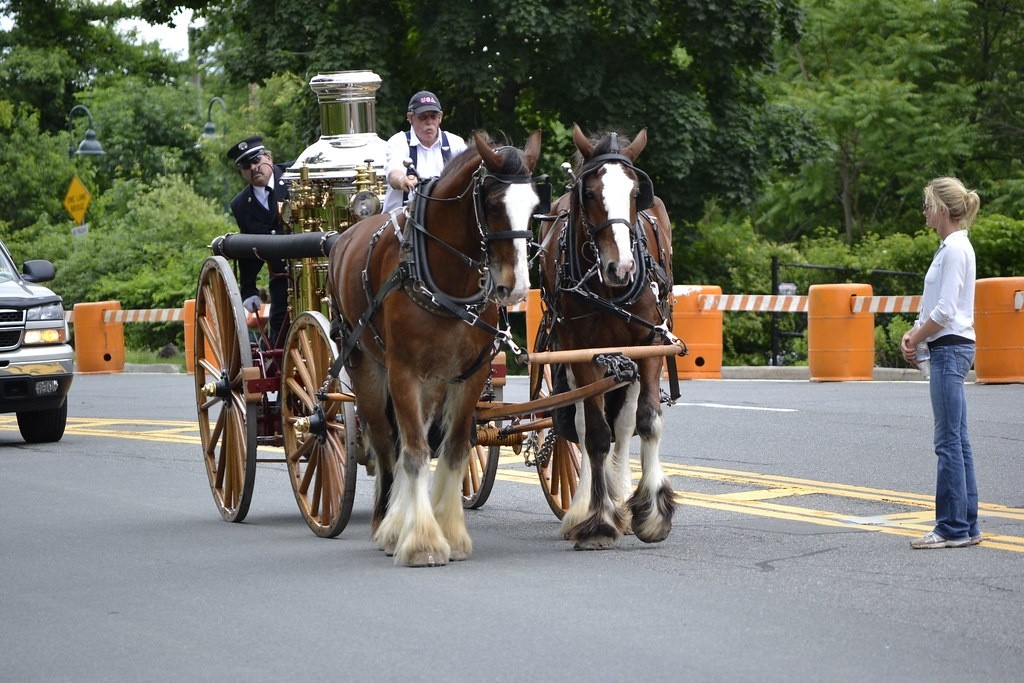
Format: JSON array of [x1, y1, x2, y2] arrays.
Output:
[[328, 128, 541, 569], [543, 122, 683, 550]]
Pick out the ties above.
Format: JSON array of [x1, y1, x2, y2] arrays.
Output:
[[265, 186, 275, 210]]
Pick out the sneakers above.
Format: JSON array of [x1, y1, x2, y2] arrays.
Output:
[[910, 530, 971, 549], [970, 535, 982, 545]]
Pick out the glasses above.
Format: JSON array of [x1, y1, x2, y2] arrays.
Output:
[[240, 156, 262, 170], [411, 114, 440, 121]]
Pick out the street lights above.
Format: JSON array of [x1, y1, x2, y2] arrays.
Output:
[[198, 96, 231, 215], [67, 104, 107, 161]]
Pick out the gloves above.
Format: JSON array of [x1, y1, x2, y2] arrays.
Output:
[[243, 295, 261, 313]]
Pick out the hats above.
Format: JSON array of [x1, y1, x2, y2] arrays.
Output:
[[227, 136, 265, 167], [408, 90, 441, 114]]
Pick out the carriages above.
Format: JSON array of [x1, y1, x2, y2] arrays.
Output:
[[195, 125, 689, 571]]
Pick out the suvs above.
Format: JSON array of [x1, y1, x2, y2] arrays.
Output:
[[0, 242, 77, 442]]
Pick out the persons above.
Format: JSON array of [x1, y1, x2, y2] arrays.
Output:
[[225, 135, 292, 352], [901, 177, 984, 550], [380, 92, 469, 214]]
